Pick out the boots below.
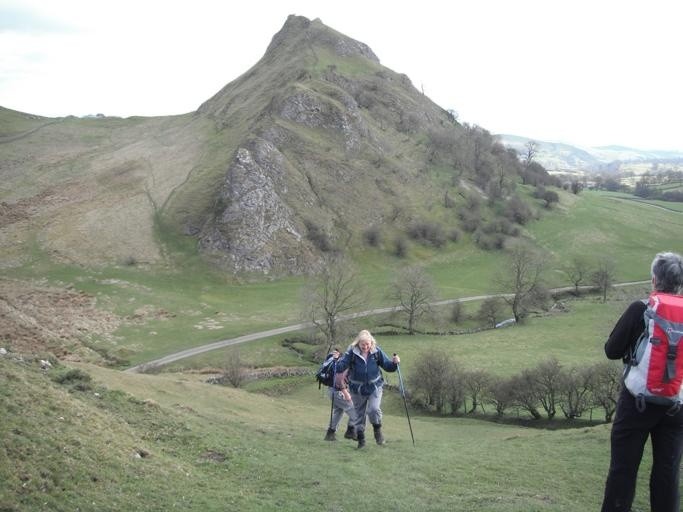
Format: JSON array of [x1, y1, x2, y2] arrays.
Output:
[[357, 431, 365, 449], [374, 424, 384, 445], [344, 425, 357, 441], [324, 428, 335, 440]]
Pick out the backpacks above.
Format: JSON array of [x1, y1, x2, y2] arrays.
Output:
[[317, 354, 334, 386], [621, 293, 683, 417]]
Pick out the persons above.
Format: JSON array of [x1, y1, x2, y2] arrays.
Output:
[[333, 329, 400, 448], [325, 367, 359, 440], [600, 251, 683, 511]]
